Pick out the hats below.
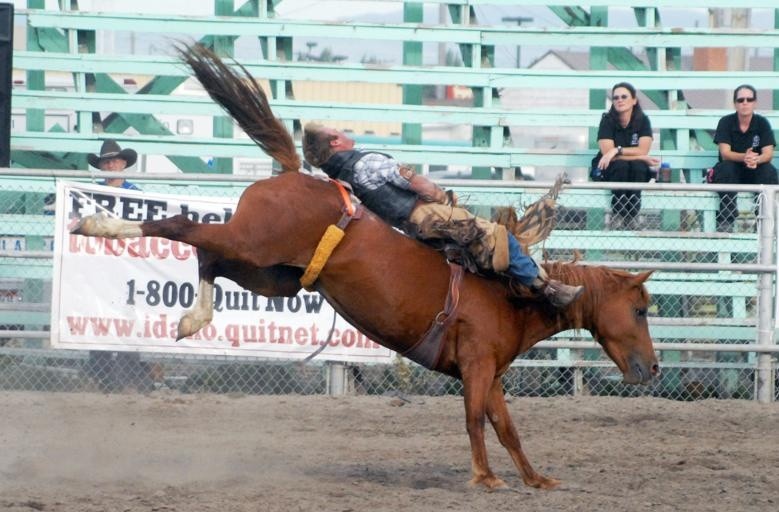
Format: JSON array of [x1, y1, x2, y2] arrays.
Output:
[[86, 139, 137, 170]]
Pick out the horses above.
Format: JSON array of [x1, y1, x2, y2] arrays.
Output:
[[68, 33, 662, 493]]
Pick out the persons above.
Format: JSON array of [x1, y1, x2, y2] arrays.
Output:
[[708, 84, 779, 234], [300, 121, 586, 310], [81, 139, 159, 397], [588, 82, 661, 230]]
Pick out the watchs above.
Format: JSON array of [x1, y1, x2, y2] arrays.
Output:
[[617, 145, 622, 156]]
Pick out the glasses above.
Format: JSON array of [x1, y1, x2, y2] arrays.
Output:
[[735, 98, 756, 104], [612, 93, 632, 100]]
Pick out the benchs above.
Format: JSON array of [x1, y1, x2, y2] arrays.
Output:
[[0, 1, 779, 396]]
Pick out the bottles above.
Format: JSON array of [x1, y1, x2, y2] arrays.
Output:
[[14, 240, 21, 251], [592, 168, 603, 180], [659, 163, 671, 183]]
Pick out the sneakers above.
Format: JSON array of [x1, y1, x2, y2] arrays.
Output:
[[546, 277, 586, 310]]
